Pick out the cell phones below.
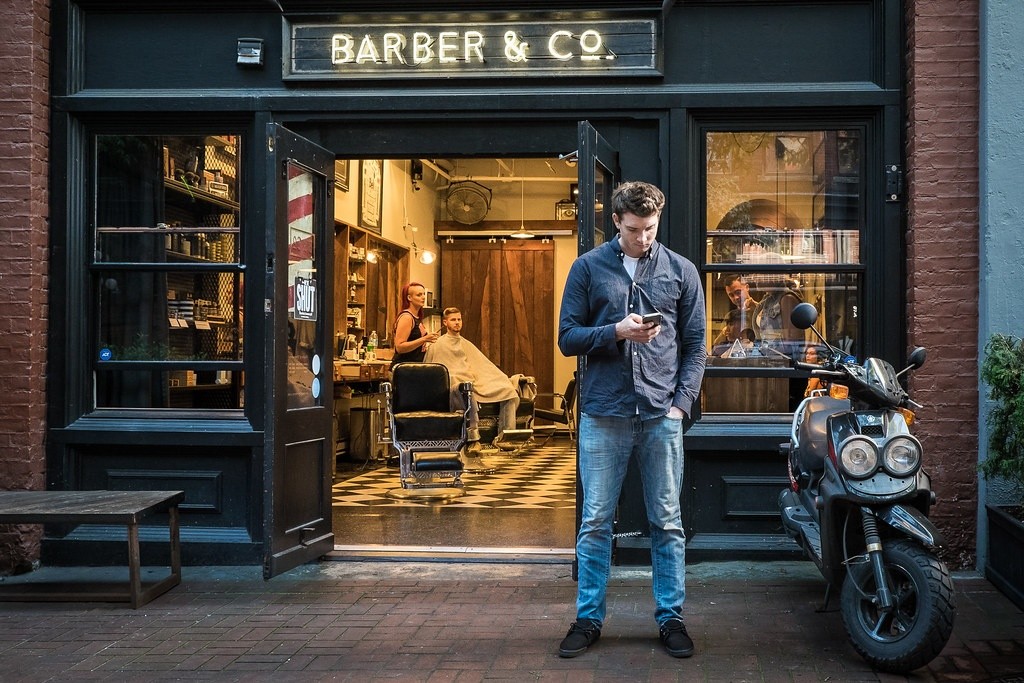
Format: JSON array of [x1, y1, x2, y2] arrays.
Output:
[[643, 313, 662, 330]]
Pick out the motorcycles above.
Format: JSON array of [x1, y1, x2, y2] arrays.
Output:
[[774, 299, 959, 674]]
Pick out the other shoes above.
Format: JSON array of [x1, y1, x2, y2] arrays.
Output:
[[492, 440, 514, 450], [468, 442, 482, 453]]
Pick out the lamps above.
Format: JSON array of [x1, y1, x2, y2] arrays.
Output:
[[408, 245, 436, 265], [511, 181, 534, 238], [367, 237, 377, 263], [234, 37, 264, 67]]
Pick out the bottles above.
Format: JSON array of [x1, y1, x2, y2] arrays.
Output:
[[369, 330, 378, 349]]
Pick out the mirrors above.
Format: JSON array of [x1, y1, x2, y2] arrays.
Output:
[[366, 236, 402, 347]]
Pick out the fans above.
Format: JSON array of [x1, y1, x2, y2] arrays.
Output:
[[446, 179, 492, 224]]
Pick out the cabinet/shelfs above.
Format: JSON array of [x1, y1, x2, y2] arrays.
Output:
[[347, 226, 367, 350], [153, 134, 243, 388]]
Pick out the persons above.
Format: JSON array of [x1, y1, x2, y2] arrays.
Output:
[[717, 254, 825, 399], [425, 304, 521, 452], [388, 282, 439, 372], [558, 183, 706, 657]]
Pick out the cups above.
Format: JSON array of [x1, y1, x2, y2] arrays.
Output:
[[345, 348, 357, 360]]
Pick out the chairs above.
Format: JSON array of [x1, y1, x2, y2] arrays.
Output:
[[535, 370, 579, 448], [459, 377, 537, 458], [379, 362, 472, 488]]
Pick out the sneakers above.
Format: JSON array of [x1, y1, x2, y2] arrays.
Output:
[[660, 621, 694, 656], [558, 618, 601, 655]]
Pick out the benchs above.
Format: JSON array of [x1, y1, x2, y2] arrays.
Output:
[[0, 491, 184, 609]]
